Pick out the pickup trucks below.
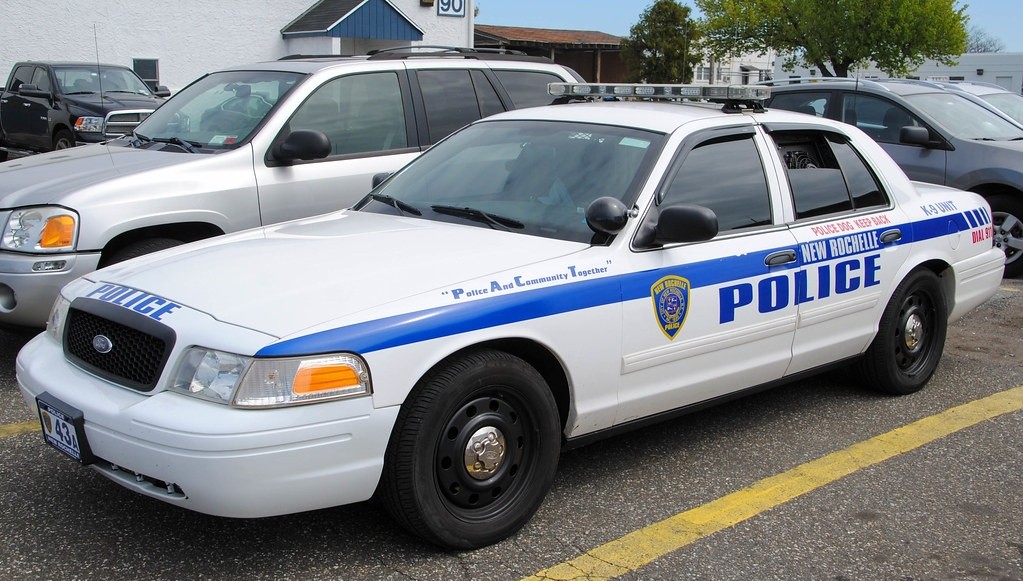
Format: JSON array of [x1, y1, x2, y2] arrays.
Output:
[[0, 59, 191, 162]]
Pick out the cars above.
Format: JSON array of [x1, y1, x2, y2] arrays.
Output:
[[200, 91, 340, 140], [745, 79, 1023, 278], [809, 70, 1022, 136], [13, 80, 1009, 547]]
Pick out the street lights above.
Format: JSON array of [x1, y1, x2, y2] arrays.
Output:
[[677, 26, 686, 83]]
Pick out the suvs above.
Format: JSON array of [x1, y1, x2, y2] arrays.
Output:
[[0, 40, 593, 327]]
